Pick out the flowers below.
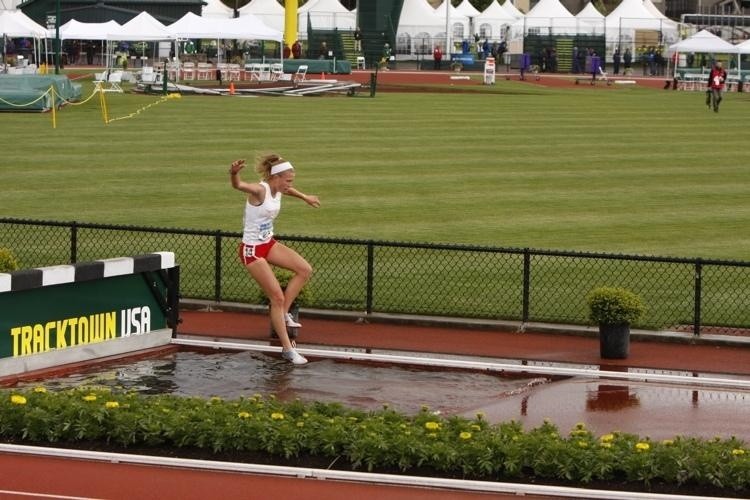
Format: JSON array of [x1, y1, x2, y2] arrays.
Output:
[[0, 385, 750, 492]]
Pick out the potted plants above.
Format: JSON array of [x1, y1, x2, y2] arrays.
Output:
[[587, 285, 649, 358], [257, 266, 314, 338]]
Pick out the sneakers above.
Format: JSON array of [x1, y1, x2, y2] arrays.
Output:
[[283, 347, 308, 365], [284, 312, 302, 328]]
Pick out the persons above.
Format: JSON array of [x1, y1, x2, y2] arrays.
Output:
[[227, 154, 322, 364], [122, 48, 128, 69], [641, 45, 664, 75], [540, 48, 548, 72], [472, 32, 507, 64], [321, 42, 330, 60], [225, 41, 232, 63], [219, 44, 224, 63], [292, 40, 301, 59], [462, 38, 469, 54], [283, 42, 291, 59], [63, 40, 81, 65], [520, 50, 530, 80], [623, 48, 632, 75], [672, 52, 680, 77], [686, 53, 694, 68], [613, 50, 620, 74], [87, 44, 96, 64], [573, 46, 597, 73], [354, 27, 363, 52], [242, 40, 251, 61], [434, 44, 442, 70], [550, 47, 557, 72], [383, 43, 392, 68], [706, 59, 727, 113]]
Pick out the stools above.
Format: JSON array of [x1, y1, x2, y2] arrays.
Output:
[[357, 57, 365, 70]]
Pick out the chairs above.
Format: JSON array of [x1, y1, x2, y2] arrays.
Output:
[[92, 58, 309, 93], [674, 72, 750, 92], [598, 67, 608, 81]]
